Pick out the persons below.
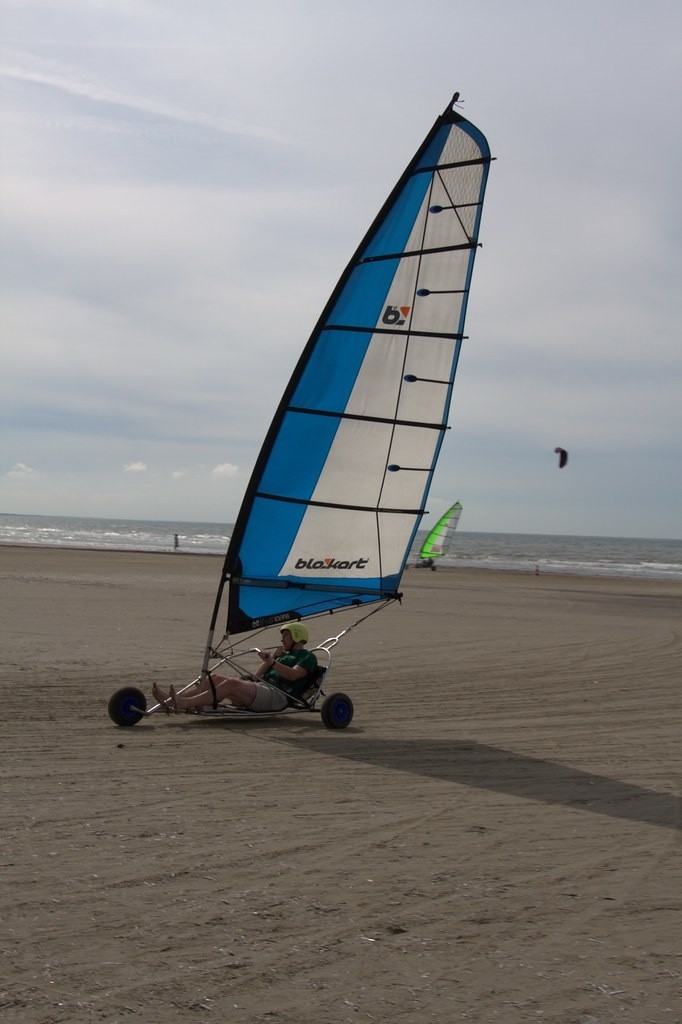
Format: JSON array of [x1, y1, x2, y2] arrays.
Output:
[[173, 533, 179, 550], [150, 622, 318, 713], [423, 557, 434, 567]]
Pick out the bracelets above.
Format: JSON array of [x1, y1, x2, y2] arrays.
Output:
[[270, 659, 276, 668]]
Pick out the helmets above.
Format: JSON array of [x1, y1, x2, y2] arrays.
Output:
[[280, 622, 308, 644]]
